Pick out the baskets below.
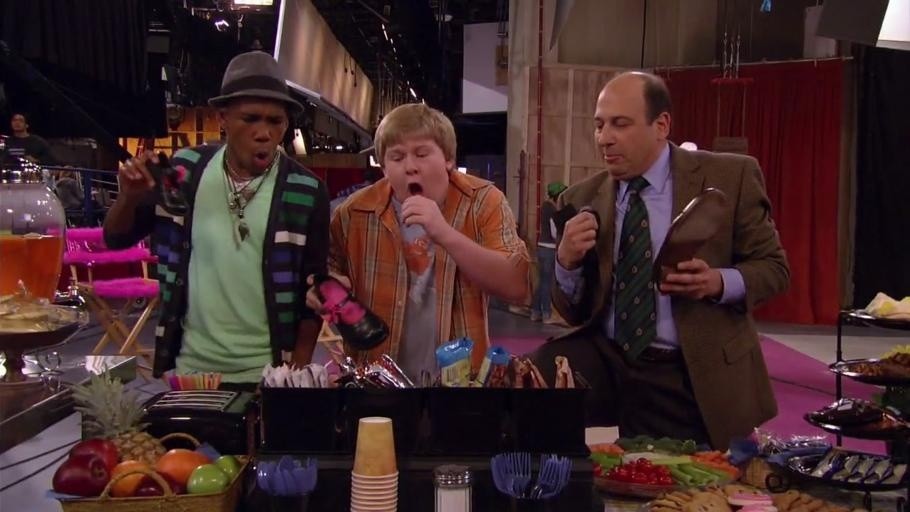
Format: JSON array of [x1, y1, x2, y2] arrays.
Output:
[[58, 432, 252, 512]]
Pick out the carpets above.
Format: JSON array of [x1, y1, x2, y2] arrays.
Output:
[[320, 331, 886, 456]]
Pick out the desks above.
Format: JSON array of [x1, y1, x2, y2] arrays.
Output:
[[0, 353, 137, 509], [141, 383, 624, 511]]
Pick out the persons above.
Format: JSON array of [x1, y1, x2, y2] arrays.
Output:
[[306, 104, 539, 389], [337, 168, 379, 198], [530, 183, 567, 325], [510, 71, 790, 453], [102, 51, 330, 392], [1, 113, 106, 228]]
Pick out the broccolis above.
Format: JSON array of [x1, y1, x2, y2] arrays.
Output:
[[616, 435, 699, 456]]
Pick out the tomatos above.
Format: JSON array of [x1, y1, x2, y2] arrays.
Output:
[[602, 457, 674, 489]]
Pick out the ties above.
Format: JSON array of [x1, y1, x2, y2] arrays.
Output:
[[613, 176, 658, 364]]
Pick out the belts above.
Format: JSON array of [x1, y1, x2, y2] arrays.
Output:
[[608, 339, 682, 363]]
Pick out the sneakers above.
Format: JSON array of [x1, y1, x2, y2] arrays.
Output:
[[530, 311, 542, 321], [543, 316, 560, 324]]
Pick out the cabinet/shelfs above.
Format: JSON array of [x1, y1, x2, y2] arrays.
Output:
[[835, 308, 909, 445]]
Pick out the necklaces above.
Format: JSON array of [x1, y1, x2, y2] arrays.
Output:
[[212, 150, 281, 241]]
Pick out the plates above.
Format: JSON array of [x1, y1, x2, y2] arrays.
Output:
[[594, 478, 735, 499]]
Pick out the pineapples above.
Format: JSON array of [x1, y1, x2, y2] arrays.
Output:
[[60, 363, 165, 467]]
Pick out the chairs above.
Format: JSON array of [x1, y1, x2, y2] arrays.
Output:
[[63, 229, 159, 377]]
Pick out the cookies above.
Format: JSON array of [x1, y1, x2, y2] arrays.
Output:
[[651, 484, 851, 512]]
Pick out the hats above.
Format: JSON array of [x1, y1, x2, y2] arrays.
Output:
[[207, 51, 305, 113], [547, 183, 568, 198]]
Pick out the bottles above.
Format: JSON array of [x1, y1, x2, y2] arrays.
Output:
[[434, 465, 474, 512]]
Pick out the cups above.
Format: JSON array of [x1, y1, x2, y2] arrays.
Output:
[[350, 417, 399, 512]]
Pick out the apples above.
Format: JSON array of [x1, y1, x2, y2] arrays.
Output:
[[54, 454, 109, 493], [188, 466, 230, 495], [217, 455, 240, 479], [69, 440, 117, 470]]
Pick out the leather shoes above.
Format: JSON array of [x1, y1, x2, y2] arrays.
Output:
[[145, 151, 191, 217], [651, 187, 729, 280], [312, 273, 389, 350]]
